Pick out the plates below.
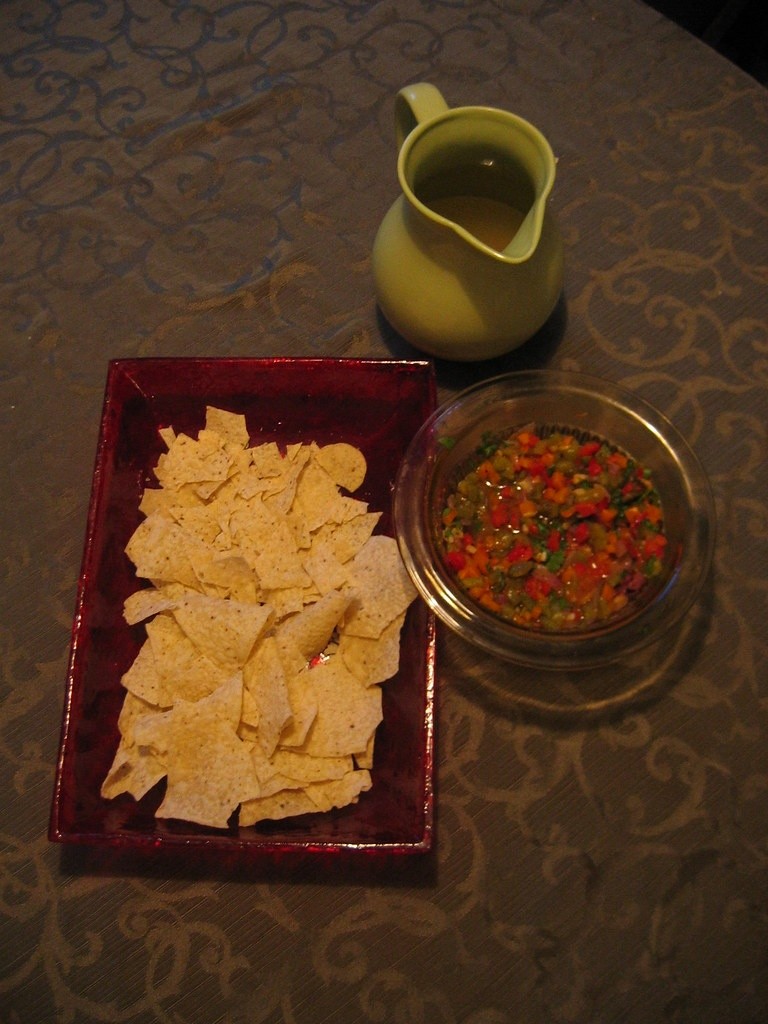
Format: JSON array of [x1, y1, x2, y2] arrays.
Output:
[[47, 359, 441, 857]]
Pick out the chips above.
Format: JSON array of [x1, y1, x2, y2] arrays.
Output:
[[99, 406, 421, 831]]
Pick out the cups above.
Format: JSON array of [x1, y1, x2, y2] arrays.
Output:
[[372, 82, 563, 358]]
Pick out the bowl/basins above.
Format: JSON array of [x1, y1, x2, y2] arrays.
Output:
[[395, 370, 713, 669]]
